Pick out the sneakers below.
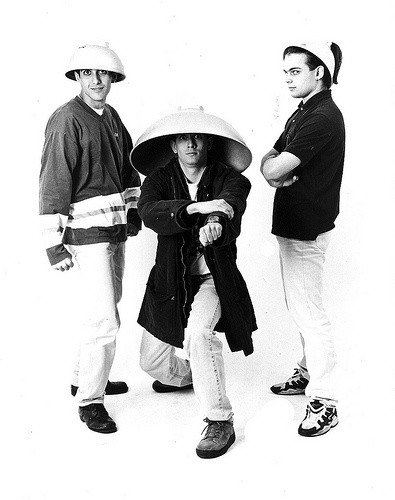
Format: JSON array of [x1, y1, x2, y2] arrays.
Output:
[[197, 418, 235, 459], [269, 367, 309, 394], [152, 379, 192, 392], [297, 401, 338, 437]]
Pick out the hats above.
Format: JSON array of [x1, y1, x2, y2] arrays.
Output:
[[65, 45, 126, 83], [129, 109, 251, 176]]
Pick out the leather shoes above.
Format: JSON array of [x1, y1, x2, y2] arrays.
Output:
[[78, 403, 116, 434], [71, 380, 129, 397]]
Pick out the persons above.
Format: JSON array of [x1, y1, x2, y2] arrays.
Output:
[[260, 33, 346, 436], [40, 41, 141, 433], [129, 107, 258, 459]]
